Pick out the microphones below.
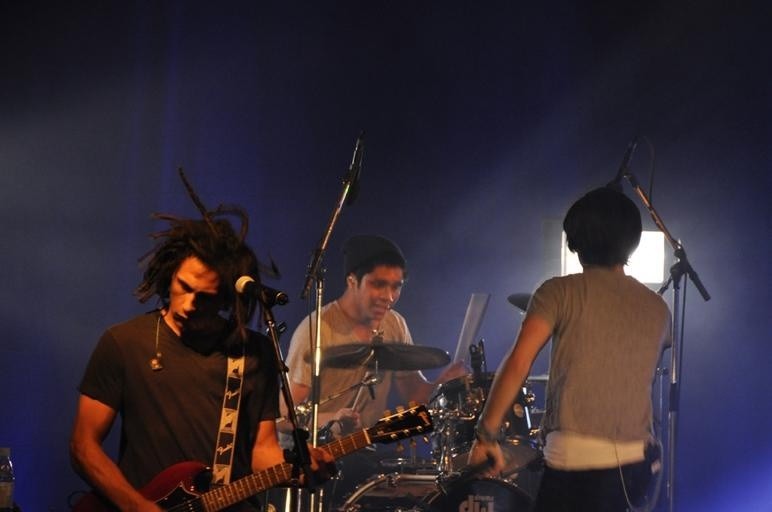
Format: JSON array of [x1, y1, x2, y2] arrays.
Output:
[[346, 144, 364, 206], [234, 275, 288, 305], [469, 339, 483, 370], [605, 135, 640, 195]]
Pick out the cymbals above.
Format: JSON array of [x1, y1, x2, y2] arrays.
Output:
[[527, 374, 550, 386], [302, 341, 451, 371], [507, 293, 534, 311]]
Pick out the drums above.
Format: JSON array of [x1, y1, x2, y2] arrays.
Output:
[[332, 459, 540, 512], [429, 371, 542, 483]]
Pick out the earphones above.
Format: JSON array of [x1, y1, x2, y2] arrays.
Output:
[[150, 358, 163, 371]]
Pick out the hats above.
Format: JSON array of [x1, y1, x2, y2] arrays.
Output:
[[343, 234, 406, 282]]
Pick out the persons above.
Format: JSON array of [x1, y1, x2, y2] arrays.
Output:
[[67, 215, 336, 512], [467, 185, 673, 511], [274, 235, 467, 511]]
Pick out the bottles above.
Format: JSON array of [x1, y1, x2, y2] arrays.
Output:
[[0, 446, 17, 511]]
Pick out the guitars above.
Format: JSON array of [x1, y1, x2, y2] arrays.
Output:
[[70, 399, 435, 512]]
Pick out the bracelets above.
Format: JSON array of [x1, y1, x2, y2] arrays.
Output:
[[474, 425, 496, 448]]
